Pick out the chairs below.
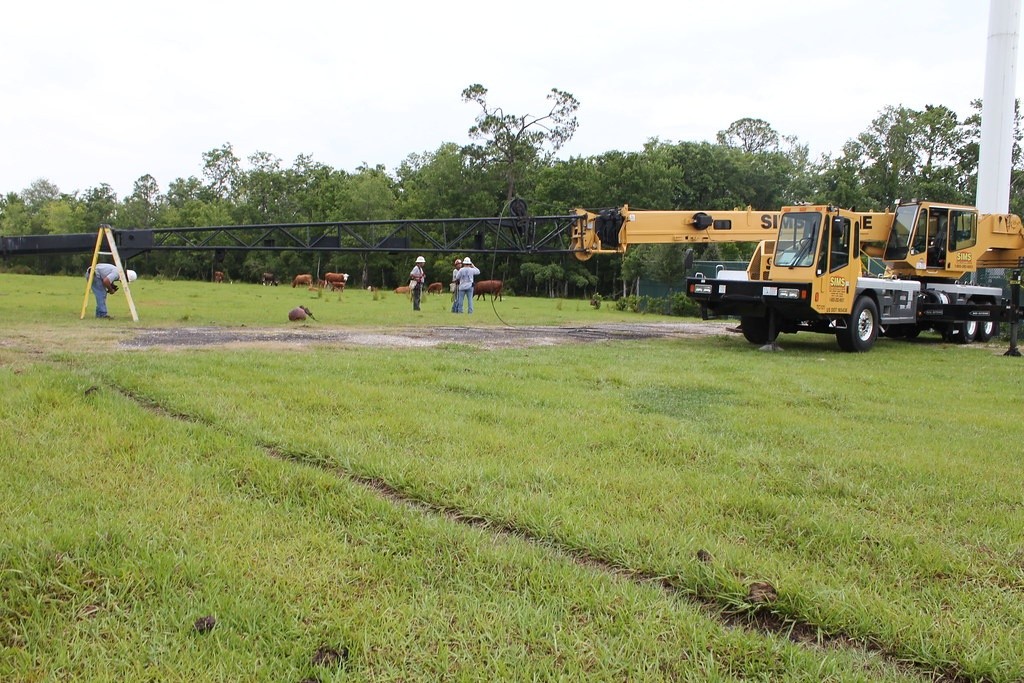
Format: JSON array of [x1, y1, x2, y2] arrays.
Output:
[[917, 224, 954, 265], [811, 230, 841, 272]]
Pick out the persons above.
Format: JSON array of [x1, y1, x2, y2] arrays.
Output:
[[85, 262, 138, 319], [451, 256, 481, 314], [410, 255, 427, 310]]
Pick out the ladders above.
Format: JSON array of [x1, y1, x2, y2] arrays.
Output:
[[78, 223, 142, 322]]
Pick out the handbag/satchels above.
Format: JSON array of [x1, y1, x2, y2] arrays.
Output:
[[408, 280, 417, 289], [449, 283, 457, 292]]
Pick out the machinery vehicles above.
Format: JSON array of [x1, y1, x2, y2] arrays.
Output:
[[0, 197, 1024, 357]]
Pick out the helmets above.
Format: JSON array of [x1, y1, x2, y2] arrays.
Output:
[[463, 257, 473, 265], [414, 255, 426, 263], [126, 269, 136, 282], [454, 259, 461, 264]]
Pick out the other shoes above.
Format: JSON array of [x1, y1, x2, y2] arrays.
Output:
[[102, 315, 114, 321]]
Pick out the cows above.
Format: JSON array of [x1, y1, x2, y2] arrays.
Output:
[[214, 271, 502, 322]]
